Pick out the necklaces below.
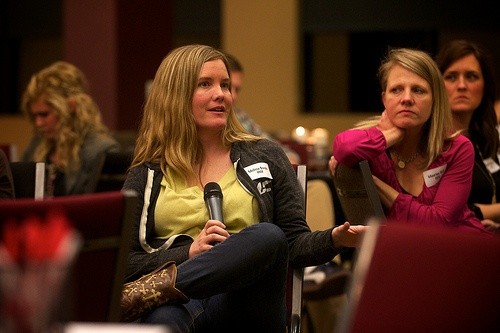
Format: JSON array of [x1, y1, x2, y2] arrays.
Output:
[[390, 146, 427, 168]]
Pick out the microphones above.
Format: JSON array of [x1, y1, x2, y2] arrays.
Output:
[[204, 182, 224, 244]]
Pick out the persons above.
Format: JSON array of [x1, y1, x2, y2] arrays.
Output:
[[220, 51, 271, 142], [432, 39, 500, 232], [120, 44, 374, 333], [328, 50, 492, 234], [23, 61, 120, 197]]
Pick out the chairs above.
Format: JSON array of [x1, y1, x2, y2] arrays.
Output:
[[0, 163, 499, 333]]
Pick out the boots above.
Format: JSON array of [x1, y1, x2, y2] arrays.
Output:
[[118, 261, 190, 322]]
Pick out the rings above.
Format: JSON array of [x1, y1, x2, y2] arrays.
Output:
[[205, 228, 209, 236]]
